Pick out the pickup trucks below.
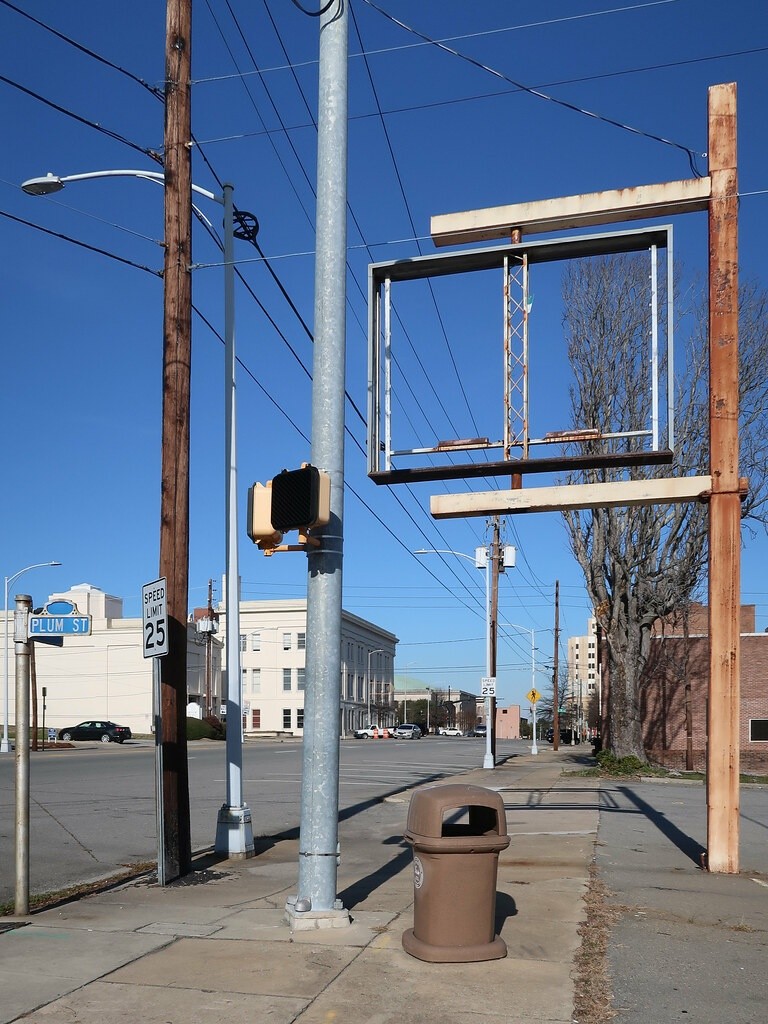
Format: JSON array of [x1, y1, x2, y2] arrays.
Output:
[[352, 724, 395, 740]]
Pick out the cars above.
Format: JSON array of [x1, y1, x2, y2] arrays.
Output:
[[393, 723, 422, 740], [59, 720, 132, 745], [416, 722, 429, 738], [545, 728, 580, 746], [439, 727, 464, 737], [474, 725, 487, 738]]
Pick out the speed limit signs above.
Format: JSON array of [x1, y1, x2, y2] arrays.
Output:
[[141, 576, 171, 659], [482, 676, 495, 698]]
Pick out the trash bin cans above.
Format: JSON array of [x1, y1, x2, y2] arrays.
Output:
[[402, 783, 512, 963]]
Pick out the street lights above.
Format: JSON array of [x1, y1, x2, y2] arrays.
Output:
[[241, 627, 280, 744], [403, 660, 417, 724], [19, 162, 256, 867], [426, 687, 431, 732], [498, 623, 539, 756], [368, 648, 385, 728], [2, 561, 64, 753], [414, 548, 495, 770]]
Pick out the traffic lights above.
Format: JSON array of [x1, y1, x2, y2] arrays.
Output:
[[268, 465, 332, 534]]
